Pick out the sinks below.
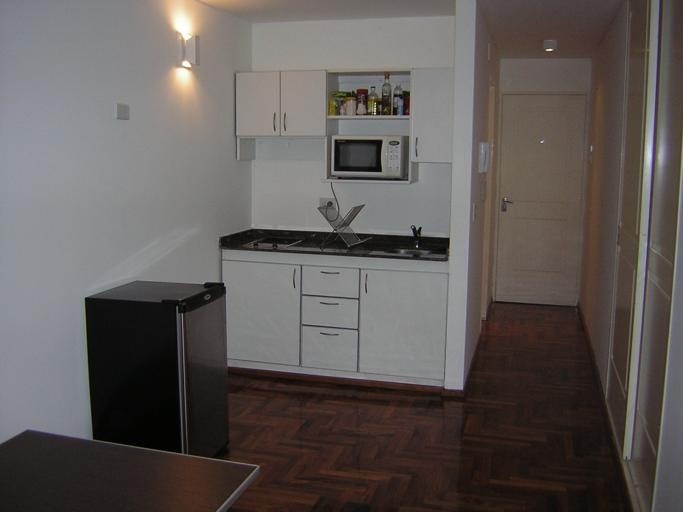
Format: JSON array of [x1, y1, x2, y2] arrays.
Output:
[[367, 248, 447, 259]]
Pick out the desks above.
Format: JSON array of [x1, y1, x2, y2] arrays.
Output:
[[0, 430, 260, 512]]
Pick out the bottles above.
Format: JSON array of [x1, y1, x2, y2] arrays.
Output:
[[382, 72, 391, 115], [377, 97, 383, 114], [355, 88, 367, 115], [344, 97, 356, 115], [366, 86, 377, 116], [393, 81, 402, 116], [404, 90, 410, 115]]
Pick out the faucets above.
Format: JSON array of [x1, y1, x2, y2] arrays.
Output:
[[410, 225, 422, 249]]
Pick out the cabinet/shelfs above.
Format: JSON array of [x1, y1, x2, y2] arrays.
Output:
[[85, 282, 228, 460], [301, 252, 359, 378], [360, 257, 448, 389], [218, 247, 301, 376], [583, 0, 683, 511], [236, 66, 453, 183]]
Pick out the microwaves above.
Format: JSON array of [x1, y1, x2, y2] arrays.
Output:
[[329, 135, 408, 179]]
